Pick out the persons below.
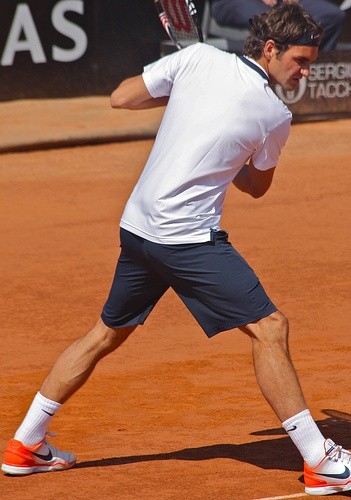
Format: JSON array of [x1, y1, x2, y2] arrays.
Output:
[[210, 0, 347, 52], [2, 3, 350, 496]]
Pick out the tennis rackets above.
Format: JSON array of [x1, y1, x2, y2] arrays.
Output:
[[156, 0, 205, 49]]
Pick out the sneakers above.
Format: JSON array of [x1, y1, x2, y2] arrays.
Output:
[[304, 438, 351, 496], [1, 439, 76, 474]]
